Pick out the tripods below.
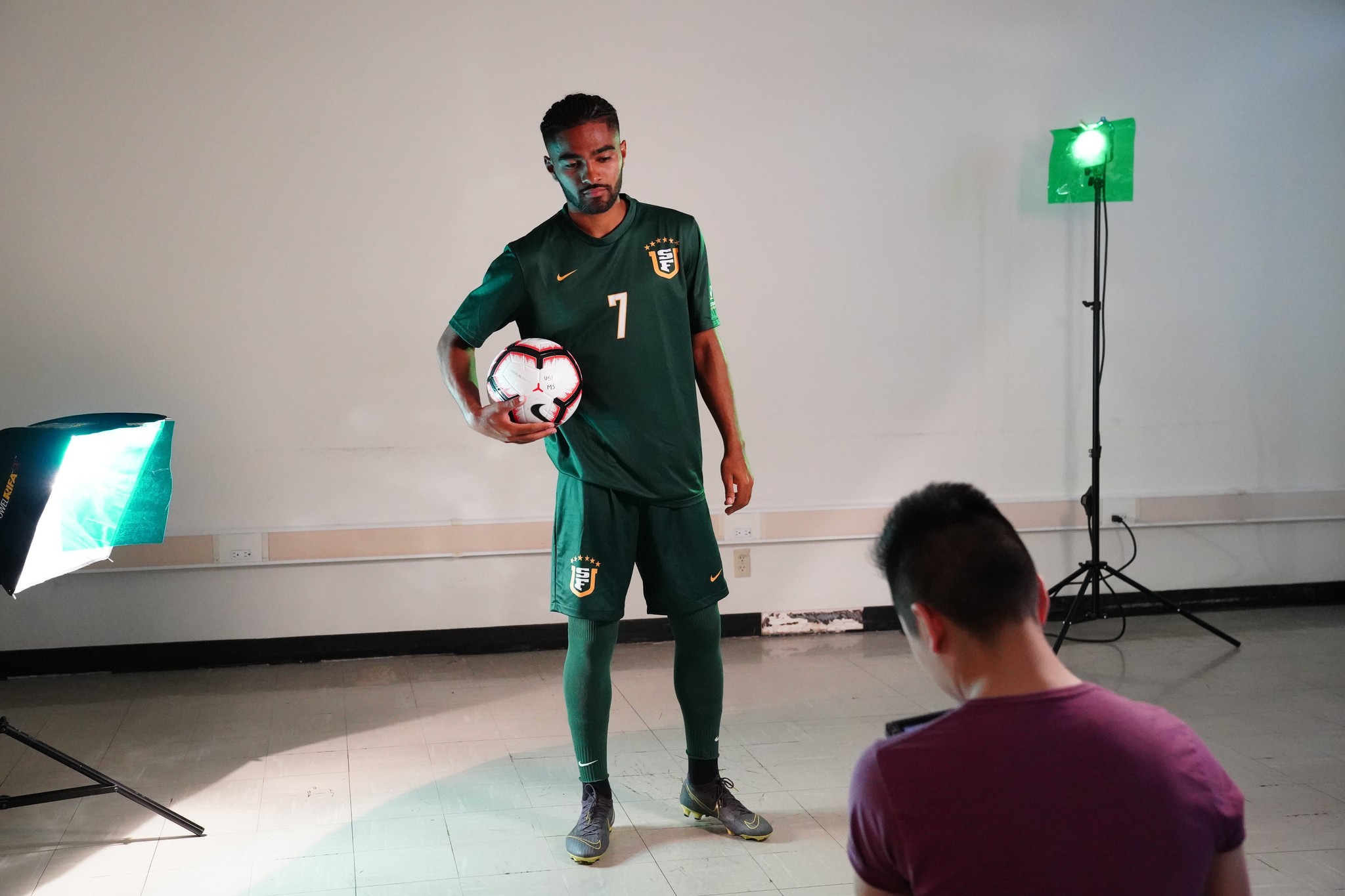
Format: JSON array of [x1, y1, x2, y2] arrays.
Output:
[[1044, 180, 1241, 658]]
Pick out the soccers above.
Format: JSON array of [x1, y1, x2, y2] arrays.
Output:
[[486, 336, 584, 428]]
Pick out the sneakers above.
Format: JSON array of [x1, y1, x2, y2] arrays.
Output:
[[680, 772, 773, 842], [565, 784, 615, 864]]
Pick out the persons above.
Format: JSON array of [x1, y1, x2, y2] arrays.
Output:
[[846, 479, 1252, 896], [436, 94, 775, 864]]
